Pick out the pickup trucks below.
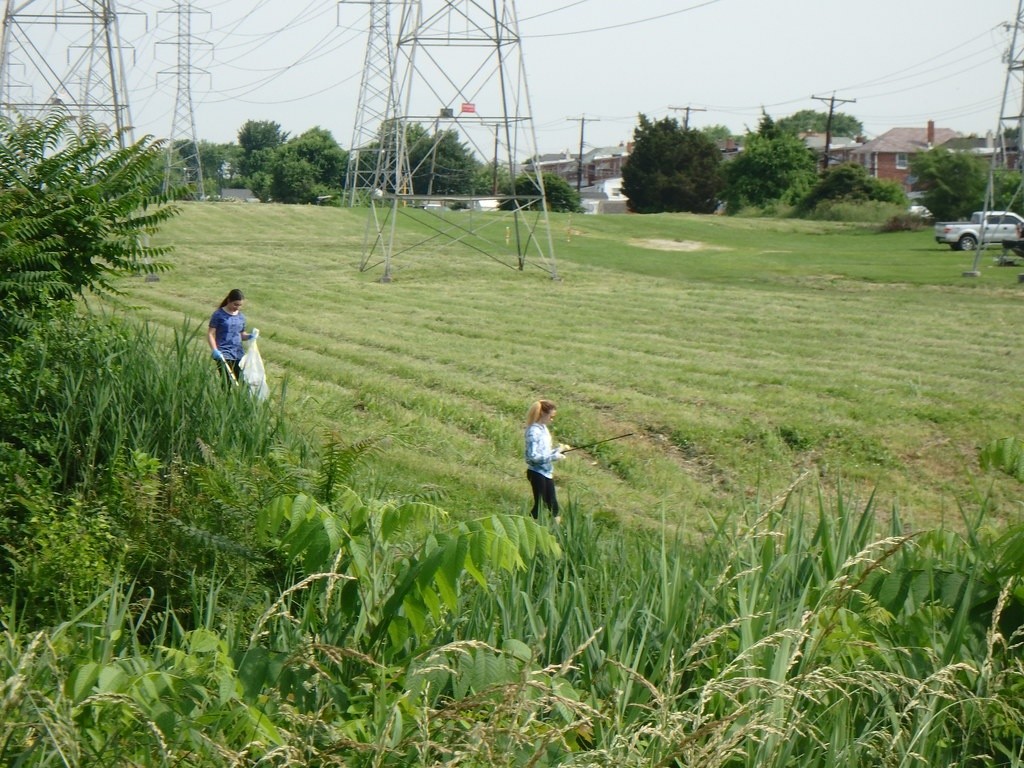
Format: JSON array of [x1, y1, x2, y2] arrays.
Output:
[[935, 210, 1024, 250]]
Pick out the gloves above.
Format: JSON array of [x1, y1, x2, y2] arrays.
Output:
[[560, 442, 571, 452], [556, 452, 566, 461], [212, 349, 222, 360]]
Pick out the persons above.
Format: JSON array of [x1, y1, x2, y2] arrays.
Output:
[[207, 288, 254, 390], [524, 399, 571, 521]]
